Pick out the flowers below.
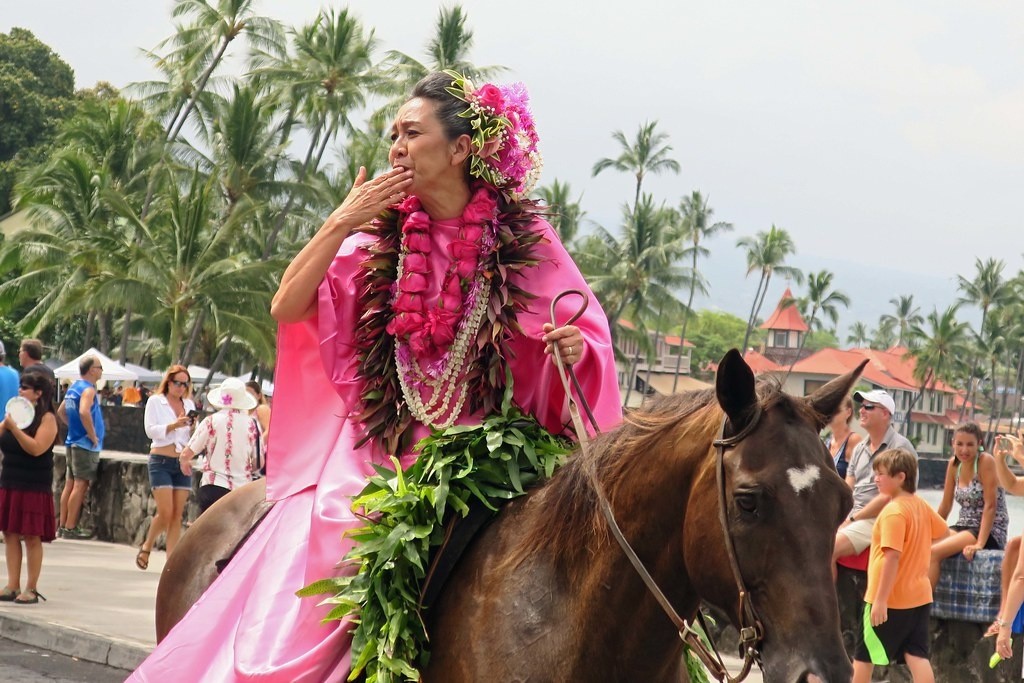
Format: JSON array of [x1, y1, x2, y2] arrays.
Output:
[[441, 66, 544, 199], [221, 395, 232, 405]]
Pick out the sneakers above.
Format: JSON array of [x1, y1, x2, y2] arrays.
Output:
[[58, 525, 92, 538]]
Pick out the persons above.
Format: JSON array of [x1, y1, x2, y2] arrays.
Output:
[[117, 67, 621, 683], [0, 338, 272, 603], [818, 388, 1024, 683]]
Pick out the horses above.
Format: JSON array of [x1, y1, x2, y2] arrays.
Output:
[[155, 344, 870, 683]]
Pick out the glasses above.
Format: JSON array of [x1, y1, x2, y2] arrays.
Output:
[[171, 379, 189, 387], [860, 403, 883, 410], [89, 366, 103, 370], [20, 385, 35, 390]]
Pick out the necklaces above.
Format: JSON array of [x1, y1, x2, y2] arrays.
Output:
[[357, 183, 547, 455]]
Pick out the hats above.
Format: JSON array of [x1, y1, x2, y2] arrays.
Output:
[[207, 377, 258, 410], [853, 389, 895, 415]]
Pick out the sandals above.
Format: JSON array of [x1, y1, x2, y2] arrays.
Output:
[[0, 587, 21, 599], [15, 590, 47, 603], [137, 546, 150, 570]]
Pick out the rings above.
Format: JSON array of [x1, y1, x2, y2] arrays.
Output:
[[1001, 645, 1006, 648], [568, 346, 573, 355]]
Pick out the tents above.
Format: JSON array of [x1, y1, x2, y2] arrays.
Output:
[[52, 347, 139, 403], [186, 365, 229, 383], [114, 359, 163, 383]]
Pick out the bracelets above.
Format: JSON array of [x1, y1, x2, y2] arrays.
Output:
[[997, 618, 1012, 627]]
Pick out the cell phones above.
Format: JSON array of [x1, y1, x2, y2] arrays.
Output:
[[186, 410, 199, 425]]
